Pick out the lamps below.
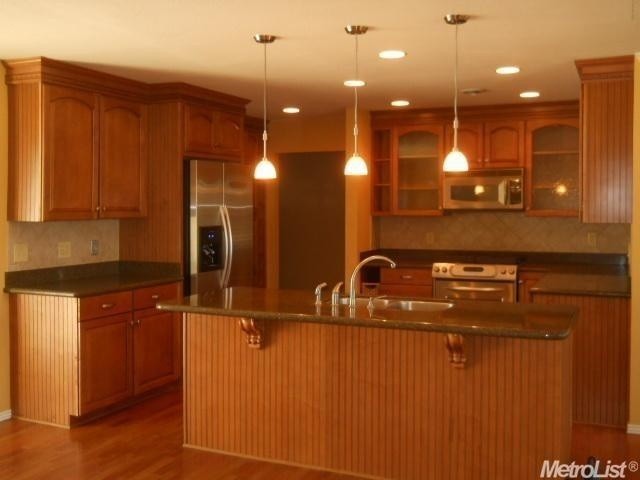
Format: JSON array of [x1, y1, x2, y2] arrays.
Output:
[[343, 24, 370, 177], [252, 34, 278, 179], [441, 14, 471, 174]]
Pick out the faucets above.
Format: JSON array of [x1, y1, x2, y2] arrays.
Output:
[[350, 255, 396, 305]]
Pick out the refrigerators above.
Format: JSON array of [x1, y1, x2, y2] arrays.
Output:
[[182, 155, 256, 301]]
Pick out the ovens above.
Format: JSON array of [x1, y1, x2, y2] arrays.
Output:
[[433, 282, 517, 307]]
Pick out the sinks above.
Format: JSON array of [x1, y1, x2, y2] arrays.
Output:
[[327, 296, 389, 305], [383, 299, 456, 313]]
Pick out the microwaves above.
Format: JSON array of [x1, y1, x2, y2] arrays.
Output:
[[440, 168, 524, 211]]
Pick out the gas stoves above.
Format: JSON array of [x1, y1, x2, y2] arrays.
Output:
[[429, 254, 521, 280]]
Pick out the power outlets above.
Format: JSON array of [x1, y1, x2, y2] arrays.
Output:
[[90, 239, 99, 256], [587, 232, 597, 248], [58, 242, 71, 258]]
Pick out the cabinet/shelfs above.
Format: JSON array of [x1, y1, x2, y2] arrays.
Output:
[[368, 109, 394, 217], [2, 57, 149, 220], [523, 99, 579, 218], [574, 54, 634, 224], [532, 293, 628, 430], [361, 267, 433, 298], [151, 84, 249, 161], [393, 109, 445, 218], [516, 270, 546, 304], [445, 103, 526, 169], [11, 282, 182, 429]]
